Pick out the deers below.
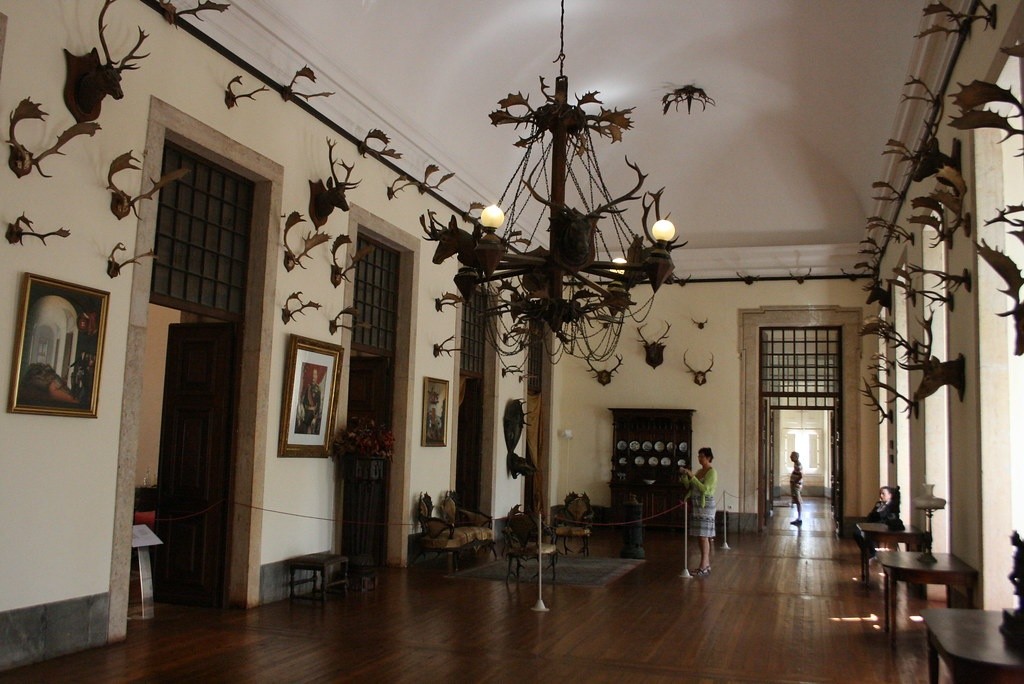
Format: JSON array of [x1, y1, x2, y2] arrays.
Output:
[[842, 1, 1024, 424], [1, 0, 717, 385], [789, 268, 812, 284], [735, 272, 761, 285]]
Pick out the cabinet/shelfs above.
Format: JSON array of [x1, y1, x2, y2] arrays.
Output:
[[607, 407, 696, 530]]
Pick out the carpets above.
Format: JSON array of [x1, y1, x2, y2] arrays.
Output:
[[445, 556, 646, 592]]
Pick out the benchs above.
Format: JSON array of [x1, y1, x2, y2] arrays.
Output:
[[413, 489, 498, 575]]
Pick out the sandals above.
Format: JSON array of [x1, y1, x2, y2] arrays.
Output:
[[706, 566, 712, 572], [690, 568, 708, 576]]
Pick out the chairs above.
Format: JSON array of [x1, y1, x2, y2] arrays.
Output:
[[551, 490, 593, 557], [503, 504, 558, 582]]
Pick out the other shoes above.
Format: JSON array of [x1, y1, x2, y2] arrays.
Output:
[[790, 520, 802, 525]]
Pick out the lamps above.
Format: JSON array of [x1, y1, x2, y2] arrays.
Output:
[[912, 483, 947, 563], [450, 0, 675, 368]]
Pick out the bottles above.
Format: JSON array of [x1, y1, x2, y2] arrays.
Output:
[[912, 483, 946, 507]]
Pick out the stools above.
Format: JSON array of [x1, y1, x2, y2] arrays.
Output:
[[286, 553, 349, 604]]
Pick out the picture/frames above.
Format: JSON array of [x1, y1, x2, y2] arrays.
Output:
[[421, 376, 449, 448], [278, 333, 344, 458], [9, 272, 111, 419]]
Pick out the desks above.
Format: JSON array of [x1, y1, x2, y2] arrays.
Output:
[[876, 551, 979, 647], [920, 608, 1024, 684], [857, 523, 923, 586]]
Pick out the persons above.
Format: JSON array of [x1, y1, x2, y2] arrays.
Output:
[[680, 447, 718, 576], [790, 451, 803, 525], [865, 486, 892, 557]]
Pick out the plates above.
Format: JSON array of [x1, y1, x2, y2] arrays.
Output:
[[619, 457, 627, 464], [649, 457, 658, 466], [678, 460, 685, 465], [667, 442, 677, 451], [642, 441, 652, 451], [617, 441, 627, 449], [635, 456, 644, 465], [661, 458, 671, 465], [679, 442, 688, 451], [629, 441, 640, 450], [654, 441, 665, 451]]
